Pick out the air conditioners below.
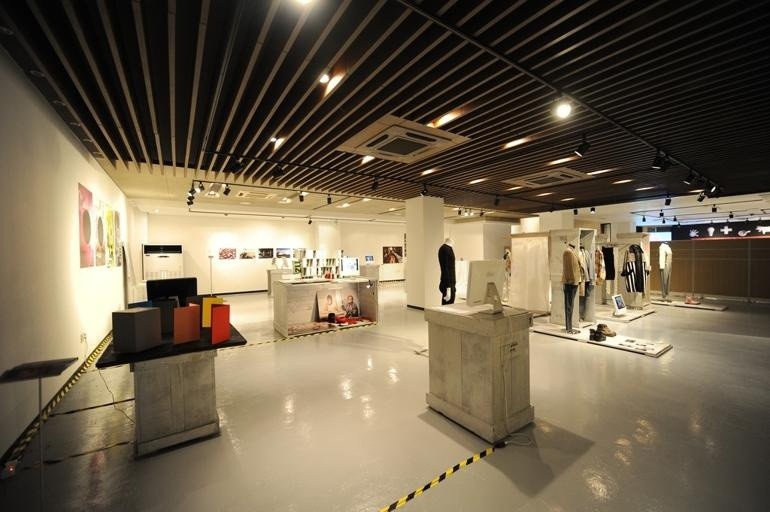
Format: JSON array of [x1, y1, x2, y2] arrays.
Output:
[[142, 242, 183, 281]]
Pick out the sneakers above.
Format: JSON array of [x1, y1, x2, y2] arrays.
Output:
[[595, 329, 606, 342], [684, 295, 701, 305], [596, 323, 617, 337], [590, 329, 596, 340]]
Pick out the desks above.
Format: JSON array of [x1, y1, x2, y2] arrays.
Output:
[[272, 273, 375, 337], [423, 299, 536, 443], [95, 292, 247, 459], [267, 268, 293, 297]]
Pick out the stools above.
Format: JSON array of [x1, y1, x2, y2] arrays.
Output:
[[0, 357, 79, 512]]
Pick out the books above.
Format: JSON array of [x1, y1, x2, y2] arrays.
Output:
[[171, 302, 200, 345], [210, 304, 231, 345], [201, 298, 223, 328]]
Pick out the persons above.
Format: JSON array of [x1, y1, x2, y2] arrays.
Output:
[[437, 237, 456, 305], [561, 243, 582, 334], [659, 241, 672, 302], [343, 295, 358, 317], [323, 295, 337, 316]]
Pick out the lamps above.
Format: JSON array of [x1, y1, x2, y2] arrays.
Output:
[[299, 191, 303, 202], [554, 100, 734, 224], [495, 196, 500, 205], [327, 194, 331, 205], [371, 177, 378, 190], [420, 184, 429, 196]]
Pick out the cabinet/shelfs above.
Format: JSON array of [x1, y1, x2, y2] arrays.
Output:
[[550, 228, 651, 327], [302, 257, 338, 280]]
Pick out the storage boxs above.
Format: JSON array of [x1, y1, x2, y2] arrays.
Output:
[[112, 306, 161, 352]]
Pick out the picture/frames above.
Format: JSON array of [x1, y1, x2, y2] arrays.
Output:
[[383, 246, 403, 264], [340, 287, 361, 318], [317, 289, 339, 319]]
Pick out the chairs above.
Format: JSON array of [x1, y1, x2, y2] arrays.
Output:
[[187, 181, 231, 207]]
